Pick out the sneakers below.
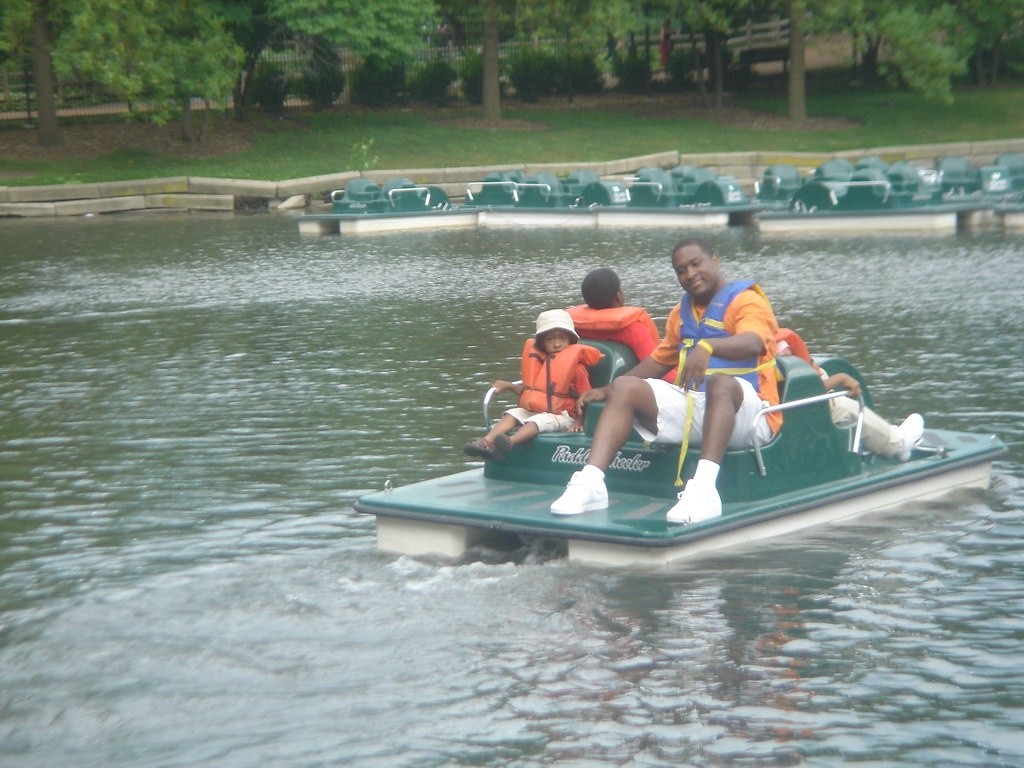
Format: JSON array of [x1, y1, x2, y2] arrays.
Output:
[[666, 477, 723, 525], [550, 469, 610, 515], [894, 412, 924, 463]]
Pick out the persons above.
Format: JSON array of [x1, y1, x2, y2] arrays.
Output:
[[463, 309, 592, 459], [576, 267, 676, 384], [776, 340, 926, 464], [550, 237, 784, 524]]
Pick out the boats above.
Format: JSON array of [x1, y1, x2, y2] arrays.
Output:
[[294, 154, 1024, 240], [352, 352, 1014, 576]]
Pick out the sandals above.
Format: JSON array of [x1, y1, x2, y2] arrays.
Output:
[[495, 433, 511, 456], [465, 439, 497, 459]]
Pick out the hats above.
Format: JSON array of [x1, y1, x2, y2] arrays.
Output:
[[535, 308, 580, 346]]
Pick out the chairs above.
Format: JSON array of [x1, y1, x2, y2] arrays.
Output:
[[329, 154, 1023, 215], [481, 337, 860, 504]]
